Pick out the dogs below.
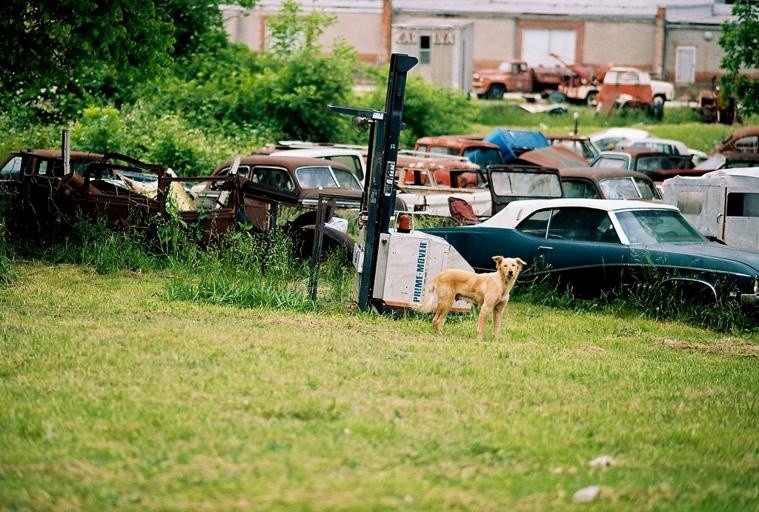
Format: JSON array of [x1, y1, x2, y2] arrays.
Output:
[[415, 255, 528, 344]]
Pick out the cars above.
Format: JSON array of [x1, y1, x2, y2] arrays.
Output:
[[408, 197, 759, 331], [558, 65, 676, 106], [1, 51, 759, 251], [202, 155, 409, 262]]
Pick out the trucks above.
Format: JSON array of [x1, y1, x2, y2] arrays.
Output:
[[470, 58, 595, 101]]
